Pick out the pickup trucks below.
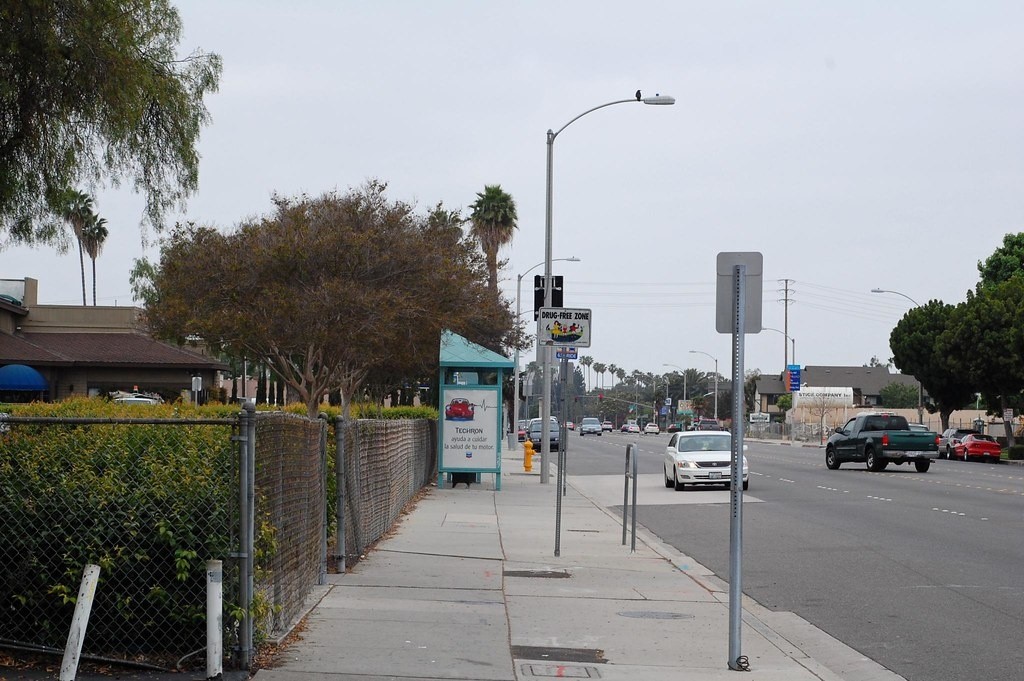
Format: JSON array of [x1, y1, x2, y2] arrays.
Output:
[[826, 414, 939, 472]]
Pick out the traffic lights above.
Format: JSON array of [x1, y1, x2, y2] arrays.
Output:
[[575, 398, 578, 402], [598, 394, 603, 402]]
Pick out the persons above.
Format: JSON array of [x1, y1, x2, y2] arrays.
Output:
[[686, 439, 699, 450], [712, 438, 730, 450]]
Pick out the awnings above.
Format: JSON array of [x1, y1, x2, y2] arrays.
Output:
[[0, 365, 50, 402]]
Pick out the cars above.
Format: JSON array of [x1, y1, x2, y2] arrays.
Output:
[[621, 424, 640, 433], [644, 423, 660, 435], [663, 430, 748, 491], [518, 416, 559, 452], [580, 417, 603, 436], [955, 434, 1001, 463], [566, 422, 574, 430], [667, 423, 697, 433]]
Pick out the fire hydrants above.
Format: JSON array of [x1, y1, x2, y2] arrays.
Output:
[[523, 441, 535, 471]]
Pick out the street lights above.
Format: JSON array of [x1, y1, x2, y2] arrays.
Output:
[[635, 372, 655, 423], [689, 351, 717, 420], [762, 328, 795, 443], [871, 289, 924, 425], [541, 95, 676, 484], [663, 364, 687, 431], [514, 258, 581, 449]]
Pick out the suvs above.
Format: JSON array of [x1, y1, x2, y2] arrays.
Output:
[[938, 428, 981, 460], [694, 419, 721, 430], [601, 421, 613, 432]]
